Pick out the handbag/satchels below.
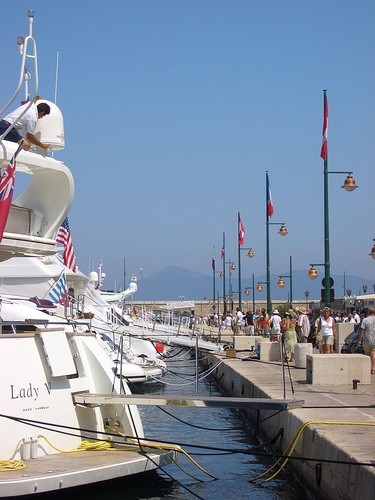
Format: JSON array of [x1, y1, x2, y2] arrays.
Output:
[[316, 331, 323, 342], [270, 321, 273, 328]]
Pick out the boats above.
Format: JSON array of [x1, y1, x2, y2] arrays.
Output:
[[0, 100, 177, 498], [58, 271, 219, 383]]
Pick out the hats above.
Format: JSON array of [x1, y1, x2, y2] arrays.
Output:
[[298, 307, 307, 314], [285, 309, 296, 315], [322, 306, 331, 311], [272, 310, 280, 314]]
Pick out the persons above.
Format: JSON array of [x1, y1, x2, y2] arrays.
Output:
[[359, 308, 375, 374], [221, 311, 233, 330], [245, 310, 253, 326], [269, 310, 282, 339], [0, 96, 50, 152], [296, 307, 311, 343], [282, 306, 297, 361], [236, 307, 243, 330], [334, 310, 365, 324], [253, 309, 260, 329], [258, 308, 269, 337], [314, 307, 337, 354]]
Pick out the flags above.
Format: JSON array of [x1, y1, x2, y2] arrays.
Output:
[[239, 220, 246, 245], [221, 249, 224, 259], [55, 216, 76, 273], [0, 154, 16, 243], [268, 187, 274, 217], [212, 256, 215, 271], [319, 99, 328, 161], [49, 270, 67, 303]]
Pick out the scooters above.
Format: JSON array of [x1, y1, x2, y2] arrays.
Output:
[[341, 319, 364, 354]]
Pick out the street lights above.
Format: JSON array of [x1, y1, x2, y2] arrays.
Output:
[[256, 222, 289, 314], [222, 261, 235, 313], [309, 172, 359, 316], [305, 290, 310, 311], [244, 287, 255, 313], [277, 276, 292, 309], [232, 248, 254, 312]]
